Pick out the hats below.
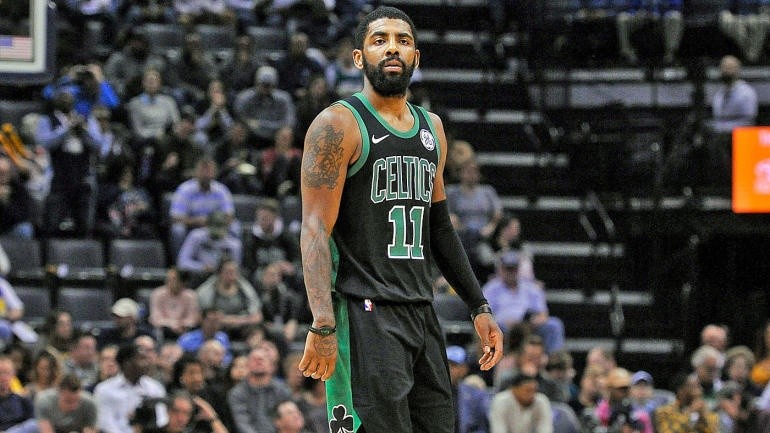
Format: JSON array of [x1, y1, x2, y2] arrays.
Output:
[[255, 66, 277, 85], [500, 252, 519, 268], [110, 298, 140, 319], [631, 371, 653, 385], [607, 367, 632, 389], [445, 346, 467, 365]]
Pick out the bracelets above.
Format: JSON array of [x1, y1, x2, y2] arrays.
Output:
[[470, 304, 493, 319], [309, 325, 338, 336]]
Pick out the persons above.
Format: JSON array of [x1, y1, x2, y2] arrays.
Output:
[[496, 36, 530, 110], [0, 0, 431, 433], [579, 324, 770, 433], [707, 57, 757, 195], [299, 7, 502, 433], [440, 140, 576, 433]]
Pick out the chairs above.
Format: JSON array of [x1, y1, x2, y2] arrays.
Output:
[[0, 0, 770, 344]]
